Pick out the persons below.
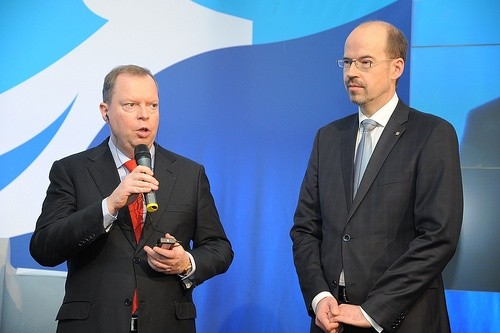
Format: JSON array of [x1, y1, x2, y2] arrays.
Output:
[[30, 65, 234, 333], [290, 21, 464, 333]]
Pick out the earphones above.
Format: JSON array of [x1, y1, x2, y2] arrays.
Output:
[[106, 113, 109, 121]]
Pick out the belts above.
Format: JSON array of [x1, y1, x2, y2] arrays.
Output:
[[338, 284, 351, 304]]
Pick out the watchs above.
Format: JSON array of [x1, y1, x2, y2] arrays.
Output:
[[179, 257, 192, 277]]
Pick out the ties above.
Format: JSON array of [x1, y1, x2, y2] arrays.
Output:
[[352, 119, 378, 200], [123, 159, 144, 317]]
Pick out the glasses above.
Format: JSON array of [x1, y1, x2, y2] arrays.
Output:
[[336, 58, 396, 69]]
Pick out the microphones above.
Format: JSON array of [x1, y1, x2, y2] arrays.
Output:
[[134, 144, 159, 213]]
